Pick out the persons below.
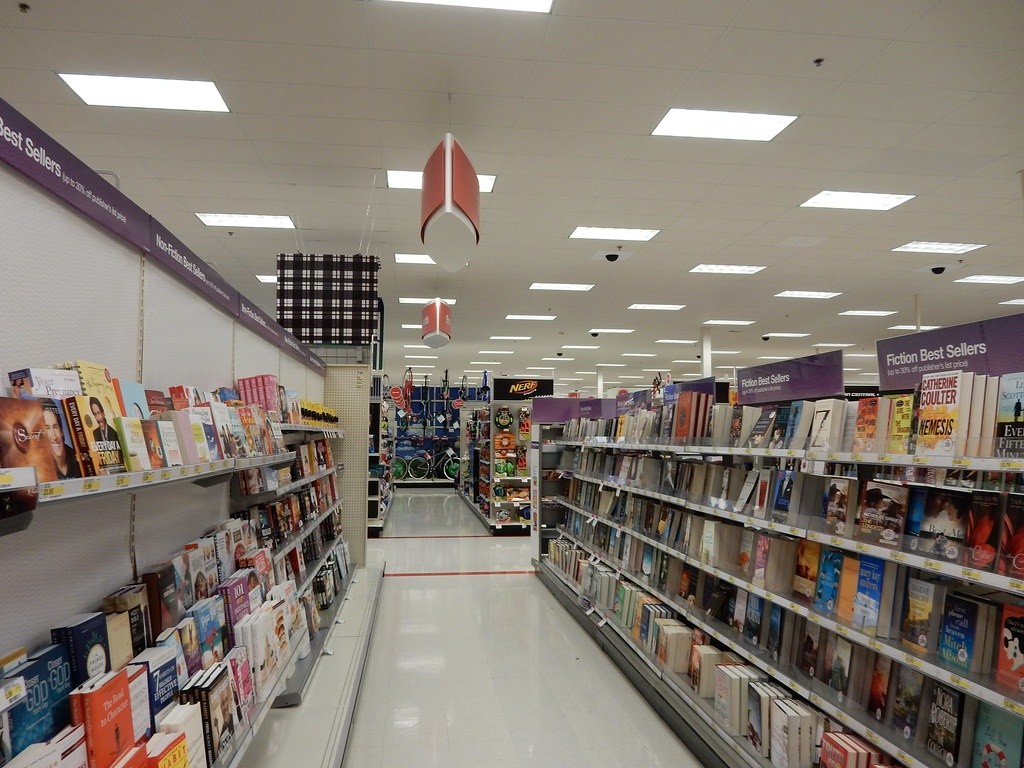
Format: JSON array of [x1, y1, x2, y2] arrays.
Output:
[[225, 533, 233, 576], [226, 424, 231, 440], [89, 397, 126, 474], [37, 398, 82, 481], [829, 484, 840, 519], [864, 488, 889, 530], [222, 426, 231, 458], [946, 493, 967, 531], [924, 491, 948, 518], [836, 486, 848, 523], [217, 685, 233, 753], [767, 428, 784, 448], [255, 467, 263, 487], [305, 496, 310, 511], [775, 471, 793, 512], [279, 387, 288, 423], [305, 456, 308, 464], [883, 497, 903, 533], [12, 379, 24, 395]]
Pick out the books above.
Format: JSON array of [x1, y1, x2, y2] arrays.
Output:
[[640, 604, 656, 654], [714, 664, 740, 737], [548, 370, 1024, 768], [0, 505, 352, 768], [747, 681, 770, 758], [0, 360, 339, 548]]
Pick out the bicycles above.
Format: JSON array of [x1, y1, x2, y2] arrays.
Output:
[[393, 455, 407, 480], [399, 368, 490, 437], [408, 445, 460, 482]]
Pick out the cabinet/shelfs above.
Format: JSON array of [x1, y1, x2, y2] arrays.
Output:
[[229, 424, 359, 709], [489, 401, 531, 537], [459, 407, 490, 526], [368, 400, 386, 539], [0, 450, 308, 768], [540, 436, 1024, 768]]
